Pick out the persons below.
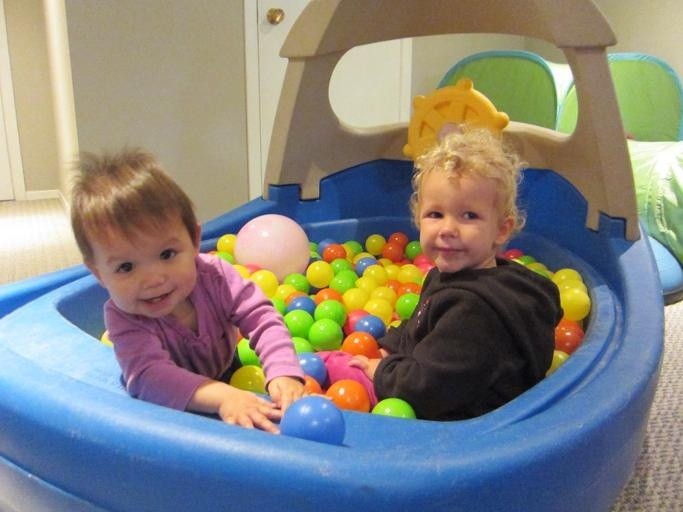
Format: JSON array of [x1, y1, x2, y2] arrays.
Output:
[[67, 147, 314, 440], [314, 125, 567, 421]]
